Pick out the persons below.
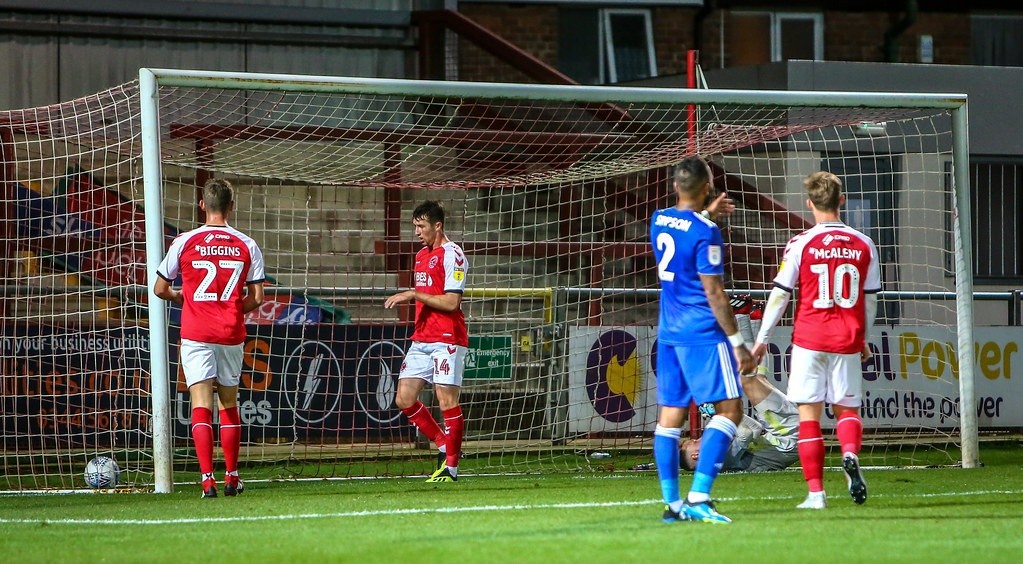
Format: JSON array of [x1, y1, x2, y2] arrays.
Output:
[[679, 293, 801, 474], [381, 200, 472, 482], [153, 177, 268, 500], [749, 170, 881, 512], [649, 157, 759, 523]]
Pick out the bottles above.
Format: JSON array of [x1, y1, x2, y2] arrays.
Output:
[[591, 452, 613, 458], [628, 463, 655, 470]]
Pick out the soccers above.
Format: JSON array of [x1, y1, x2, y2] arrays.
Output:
[[84, 456, 120, 489]]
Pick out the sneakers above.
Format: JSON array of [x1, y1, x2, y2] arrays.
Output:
[[678, 499, 731, 525], [201, 485, 218, 499], [662, 505, 677, 522], [795, 491, 827, 509], [750, 301, 765, 319], [727, 294, 753, 315], [425, 467, 458, 483], [431, 451, 462, 478], [842, 452, 867, 505], [224, 481, 244, 497]]
